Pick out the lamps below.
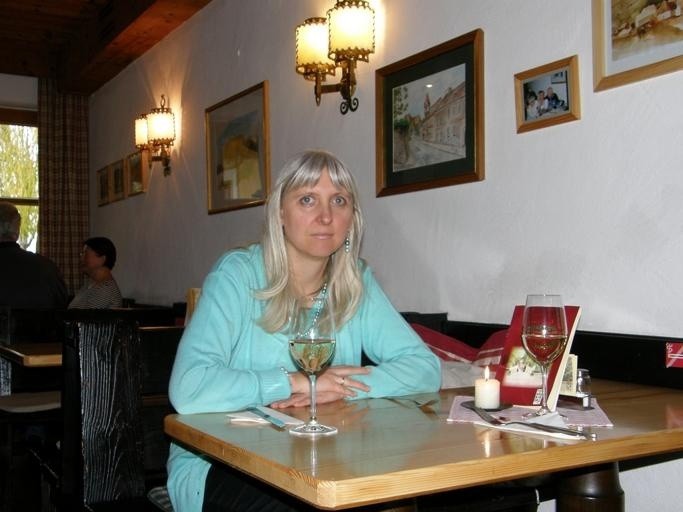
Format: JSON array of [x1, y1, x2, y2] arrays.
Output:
[[132, 94, 177, 178], [293, 0, 376, 115]]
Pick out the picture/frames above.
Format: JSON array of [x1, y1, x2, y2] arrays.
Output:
[[108, 157, 126, 202], [591, 0, 683, 93], [373, 28, 486, 200], [126, 147, 149, 198], [95, 163, 114, 207], [513, 54, 581, 135], [204, 79, 272, 216]]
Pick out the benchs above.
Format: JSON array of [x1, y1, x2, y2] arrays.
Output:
[[30, 305, 187, 512], [112, 319, 216, 510]]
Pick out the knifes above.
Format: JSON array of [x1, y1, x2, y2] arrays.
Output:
[[250, 409, 285, 430]]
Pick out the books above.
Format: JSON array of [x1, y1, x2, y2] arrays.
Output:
[[495, 305, 583, 411]]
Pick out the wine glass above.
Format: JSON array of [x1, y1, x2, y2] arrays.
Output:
[[524, 293, 568, 421], [287, 298, 338, 437]]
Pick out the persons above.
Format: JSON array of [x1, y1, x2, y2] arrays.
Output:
[[67, 237, 122, 308], [528, 96, 539, 121], [527, 88, 536, 101], [537, 90, 549, 116], [0, 202, 68, 344], [545, 87, 558, 110]]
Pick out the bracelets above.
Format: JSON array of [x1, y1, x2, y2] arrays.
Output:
[[280, 366, 293, 398]]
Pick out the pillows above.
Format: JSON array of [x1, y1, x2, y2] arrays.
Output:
[[409, 319, 480, 365], [470, 328, 509, 380]]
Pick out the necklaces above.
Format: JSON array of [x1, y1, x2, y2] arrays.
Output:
[[288, 281, 327, 337]]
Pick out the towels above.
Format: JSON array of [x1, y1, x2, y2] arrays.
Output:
[[502, 412, 586, 442], [228, 406, 306, 427]]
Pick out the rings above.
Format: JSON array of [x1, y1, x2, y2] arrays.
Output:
[[166, 149, 441, 512], [342, 376, 345, 386]]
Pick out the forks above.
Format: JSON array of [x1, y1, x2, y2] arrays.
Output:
[[473, 407, 596, 440]]
